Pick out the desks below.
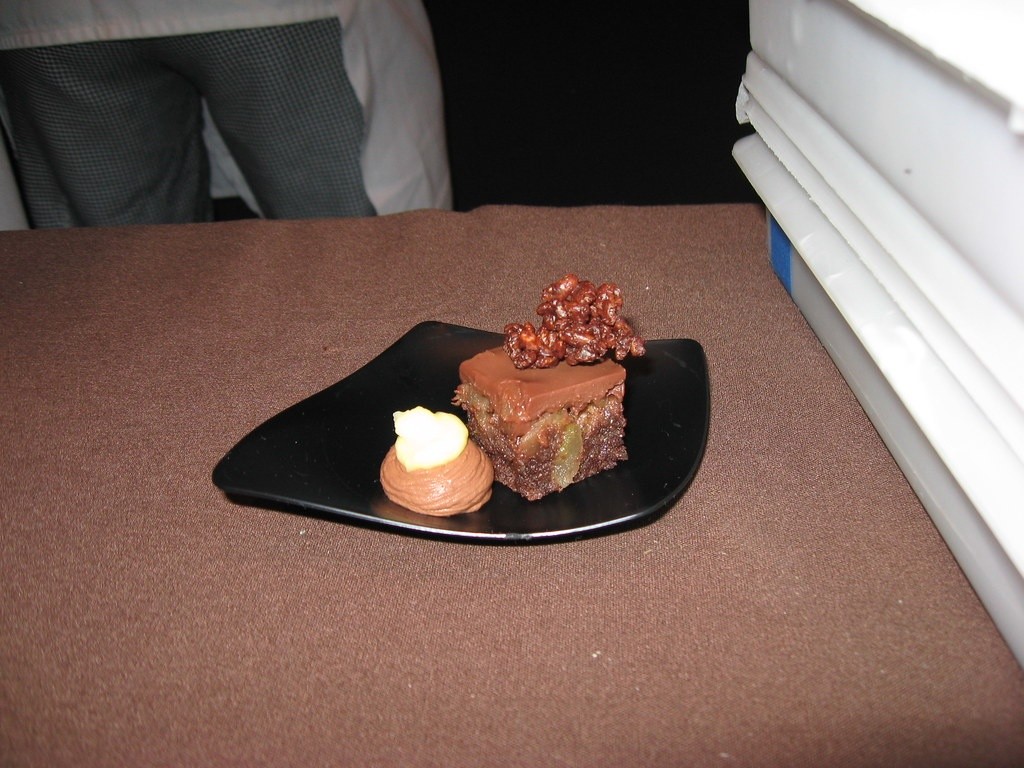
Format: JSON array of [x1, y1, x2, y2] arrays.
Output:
[[0, 202, 1024, 768]]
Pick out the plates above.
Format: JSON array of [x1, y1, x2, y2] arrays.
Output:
[[212, 320, 711, 547]]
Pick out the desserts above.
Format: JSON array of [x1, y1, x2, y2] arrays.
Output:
[[380, 273, 646, 517]]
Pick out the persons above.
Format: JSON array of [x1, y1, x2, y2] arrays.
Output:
[[0, 0, 450, 230]]
